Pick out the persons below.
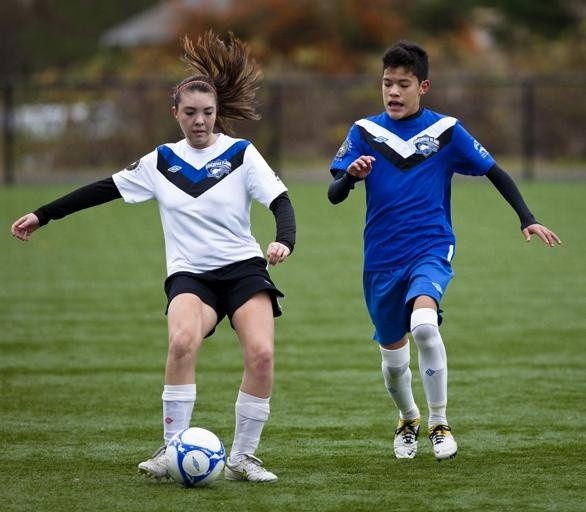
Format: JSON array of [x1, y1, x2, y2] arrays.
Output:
[[10, 31, 295, 483], [328, 39, 562, 460]]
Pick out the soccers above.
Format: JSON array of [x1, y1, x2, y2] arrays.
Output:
[[166, 428, 226, 487]]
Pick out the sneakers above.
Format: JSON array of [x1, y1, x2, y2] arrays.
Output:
[[394, 417, 422, 458], [137, 446, 176, 482], [427, 424, 458, 463], [225, 452, 279, 483]]
